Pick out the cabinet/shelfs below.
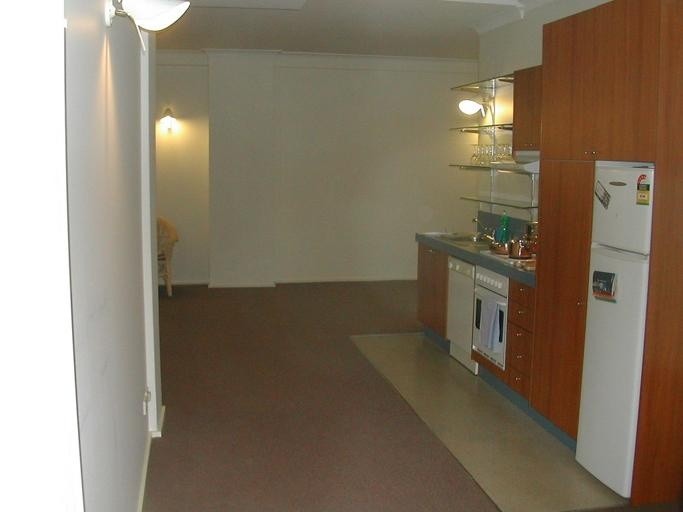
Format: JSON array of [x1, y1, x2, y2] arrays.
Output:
[[412, 0, 683, 512]]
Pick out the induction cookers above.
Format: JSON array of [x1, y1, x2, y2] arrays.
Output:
[[474, 248, 536, 297]]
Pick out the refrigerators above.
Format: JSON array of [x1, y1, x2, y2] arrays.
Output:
[[573, 161, 657, 499]]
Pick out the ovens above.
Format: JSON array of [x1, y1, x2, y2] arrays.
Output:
[[472, 284, 507, 370]]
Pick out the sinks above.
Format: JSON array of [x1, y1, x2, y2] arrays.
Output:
[[435, 232, 489, 243], [456, 243, 492, 254]]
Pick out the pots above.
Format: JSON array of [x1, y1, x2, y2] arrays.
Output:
[[507, 239, 532, 259], [481, 233, 509, 254]]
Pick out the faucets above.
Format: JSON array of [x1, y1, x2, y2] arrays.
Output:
[[471, 217, 495, 244]]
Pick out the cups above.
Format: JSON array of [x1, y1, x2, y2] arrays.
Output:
[[471, 143, 512, 168]]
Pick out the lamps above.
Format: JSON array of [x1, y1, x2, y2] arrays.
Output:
[[103, 0, 191, 53], [458, 98, 494, 125], [158, 107, 183, 137]]
